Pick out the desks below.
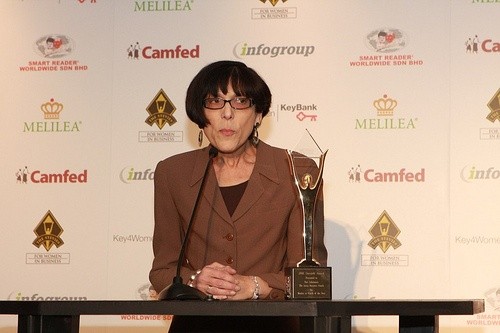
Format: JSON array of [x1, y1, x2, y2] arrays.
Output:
[[0, 299, 487, 333]]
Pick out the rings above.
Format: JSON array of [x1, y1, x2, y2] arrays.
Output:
[[207, 287, 212, 292]]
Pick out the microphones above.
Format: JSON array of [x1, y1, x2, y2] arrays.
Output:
[[158, 147, 218, 301]]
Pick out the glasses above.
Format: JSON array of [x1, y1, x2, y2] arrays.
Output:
[[202, 98, 257, 110]]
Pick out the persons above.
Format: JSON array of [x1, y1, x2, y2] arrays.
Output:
[[149, 60, 327, 333]]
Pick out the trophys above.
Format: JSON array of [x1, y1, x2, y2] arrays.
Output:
[[284, 149, 332, 300]]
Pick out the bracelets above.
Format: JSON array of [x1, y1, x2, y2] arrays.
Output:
[[186, 269, 202, 288], [251, 276, 259, 300]]
